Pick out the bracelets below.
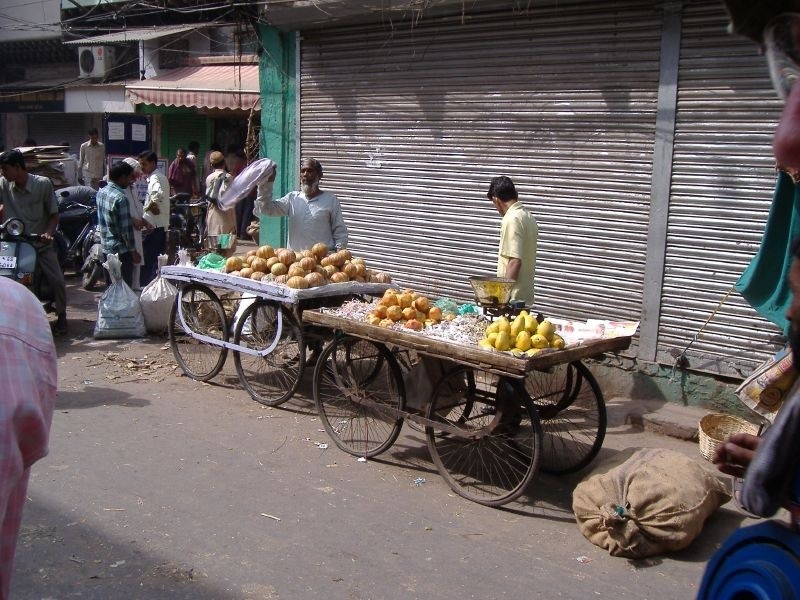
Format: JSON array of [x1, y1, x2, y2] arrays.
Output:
[[171, 187, 175, 188]]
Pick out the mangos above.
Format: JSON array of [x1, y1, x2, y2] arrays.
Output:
[[479, 310, 565, 356]]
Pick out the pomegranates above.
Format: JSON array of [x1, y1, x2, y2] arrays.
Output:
[[365, 289, 441, 331]]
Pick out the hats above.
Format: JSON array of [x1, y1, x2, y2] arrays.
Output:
[[210, 151, 224, 164]]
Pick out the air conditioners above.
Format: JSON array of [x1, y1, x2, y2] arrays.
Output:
[[78, 46, 115, 77]]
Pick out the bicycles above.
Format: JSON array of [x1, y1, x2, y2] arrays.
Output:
[[168, 192, 206, 249]]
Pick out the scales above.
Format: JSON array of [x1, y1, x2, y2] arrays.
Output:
[[468, 275, 536, 322]]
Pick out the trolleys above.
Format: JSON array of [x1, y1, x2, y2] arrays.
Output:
[[157, 265, 633, 503]]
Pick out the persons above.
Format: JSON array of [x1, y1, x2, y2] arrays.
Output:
[[480, 176, 539, 415], [714, 1, 800, 530], [0, 150, 68, 337], [96, 150, 170, 296], [253, 158, 349, 367], [168, 143, 257, 259], [78, 128, 107, 191], [0, 274, 57, 599]]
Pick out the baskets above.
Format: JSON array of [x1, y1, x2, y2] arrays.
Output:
[[699, 413, 758, 462], [182, 288, 240, 329]]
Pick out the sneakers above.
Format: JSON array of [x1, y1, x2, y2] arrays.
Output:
[[55, 312, 67, 332]]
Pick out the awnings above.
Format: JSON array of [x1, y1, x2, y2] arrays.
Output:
[[126, 55, 261, 111]]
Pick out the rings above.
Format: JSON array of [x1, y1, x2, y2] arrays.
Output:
[[138, 226, 140, 229]]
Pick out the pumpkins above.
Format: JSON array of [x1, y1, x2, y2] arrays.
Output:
[[226, 243, 392, 289]]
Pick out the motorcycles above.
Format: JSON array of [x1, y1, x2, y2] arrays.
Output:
[[0, 218, 56, 315], [57, 202, 106, 290]]
